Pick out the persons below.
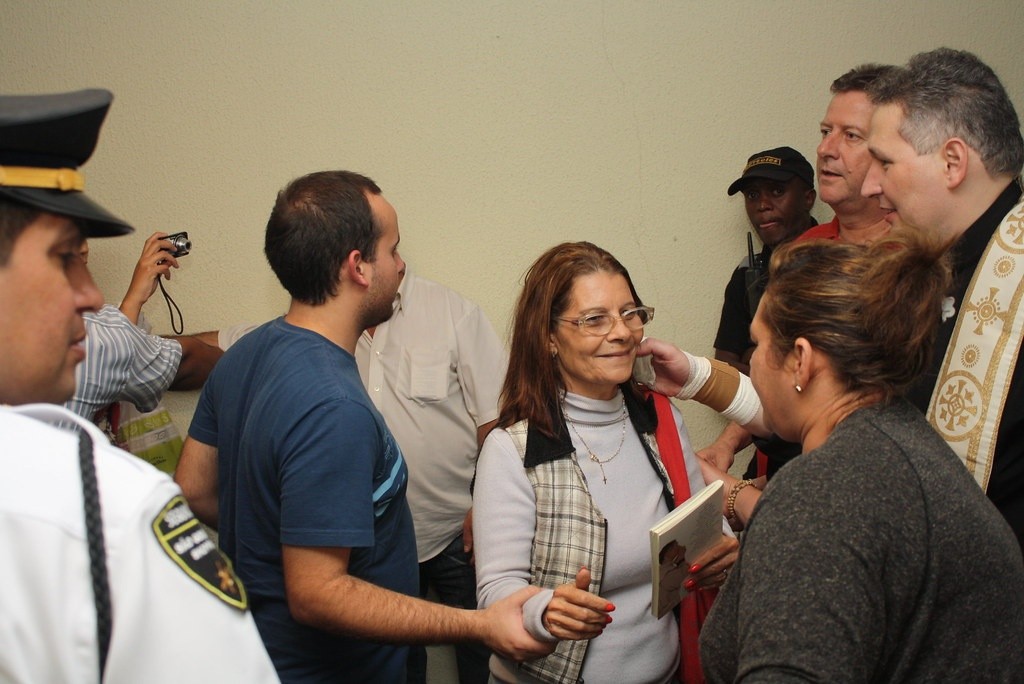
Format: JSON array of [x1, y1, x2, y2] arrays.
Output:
[[698, 222, 1024, 684], [0, 89, 286, 684], [694, 64, 915, 479], [713, 146, 819, 480], [474, 241, 735, 684], [174, 171, 555, 684], [190, 269, 510, 684], [657, 541, 690, 619], [51, 232, 226, 433], [640, 48, 1024, 546]]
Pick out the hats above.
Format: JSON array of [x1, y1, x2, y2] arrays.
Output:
[[0, 89, 133, 238], [727, 146, 814, 196]]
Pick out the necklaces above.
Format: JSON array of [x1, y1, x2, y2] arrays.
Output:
[[559, 395, 626, 485]]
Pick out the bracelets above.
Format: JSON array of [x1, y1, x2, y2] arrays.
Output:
[[727, 480, 756, 520]]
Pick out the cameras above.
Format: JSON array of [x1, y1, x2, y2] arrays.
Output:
[[158, 232, 192, 264]]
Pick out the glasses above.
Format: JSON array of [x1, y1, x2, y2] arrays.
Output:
[[555, 306, 655, 337]]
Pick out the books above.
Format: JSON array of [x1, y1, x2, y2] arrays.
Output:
[[649, 479, 725, 620]]
